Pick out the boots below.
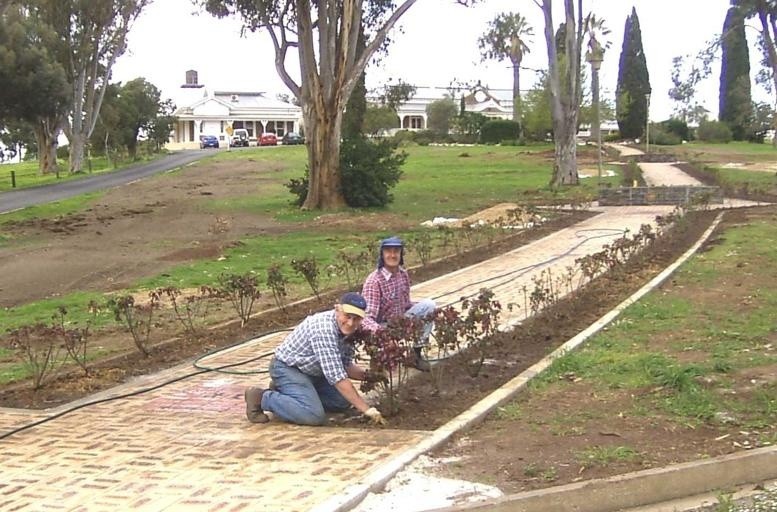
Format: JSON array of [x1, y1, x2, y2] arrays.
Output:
[[245, 386, 270, 423], [410, 346, 431, 371]]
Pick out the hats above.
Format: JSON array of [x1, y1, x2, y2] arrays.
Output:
[[378, 238, 403, 268], [340, 291, 366, 317]]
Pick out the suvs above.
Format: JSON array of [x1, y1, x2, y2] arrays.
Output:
[[229, 128, 249, 147]]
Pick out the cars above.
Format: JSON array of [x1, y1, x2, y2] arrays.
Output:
[[200, 135, 220, 148], [281, 132, 302, 145], [257, 133, 278, 145]]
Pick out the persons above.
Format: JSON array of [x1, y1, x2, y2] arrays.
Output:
[[359, 236, 437, 370], [244, 293, 392, 427]]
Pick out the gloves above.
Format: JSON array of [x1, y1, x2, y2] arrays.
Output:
[[365, 407, 388, 425], [364, 371, 388, 384]]
